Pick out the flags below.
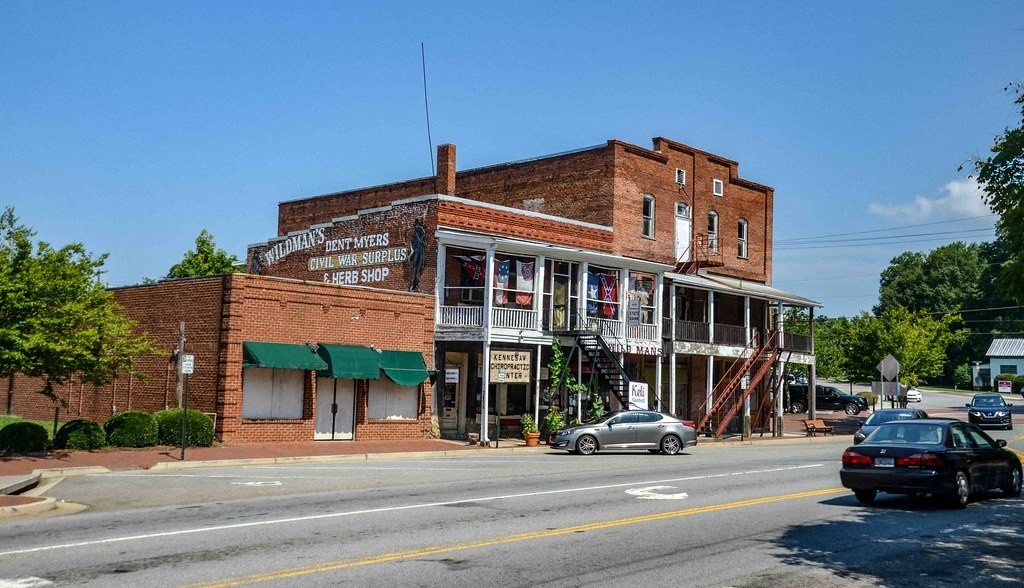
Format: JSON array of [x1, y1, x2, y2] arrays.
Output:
[[453, 255, 617, 316]]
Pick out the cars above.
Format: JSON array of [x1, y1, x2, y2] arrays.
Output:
[[853, 409, 940, 447], [965, 394, 1013, 430], [840, 417, 1023, 510], [786, 374, 809, 384], [887, 386, 922, 403], [548, 409, 697, 455]]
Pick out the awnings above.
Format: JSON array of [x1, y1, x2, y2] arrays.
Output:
[[243, 340, 439, 386]]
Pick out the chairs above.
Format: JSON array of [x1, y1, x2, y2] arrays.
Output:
[[892, 414, 900, 421], [904, 429, 920, 442], [953, 434, 965, 447]]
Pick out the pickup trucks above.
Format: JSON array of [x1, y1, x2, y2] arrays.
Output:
[[782, 383, 870, 415]]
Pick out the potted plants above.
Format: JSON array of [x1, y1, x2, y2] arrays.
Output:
[[521, 413, 566, 447]]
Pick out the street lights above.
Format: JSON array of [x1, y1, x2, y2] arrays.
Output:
[[848, 344, 853, 395]]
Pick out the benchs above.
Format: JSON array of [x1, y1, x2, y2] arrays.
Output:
[[804, 419, 834, 437]]
